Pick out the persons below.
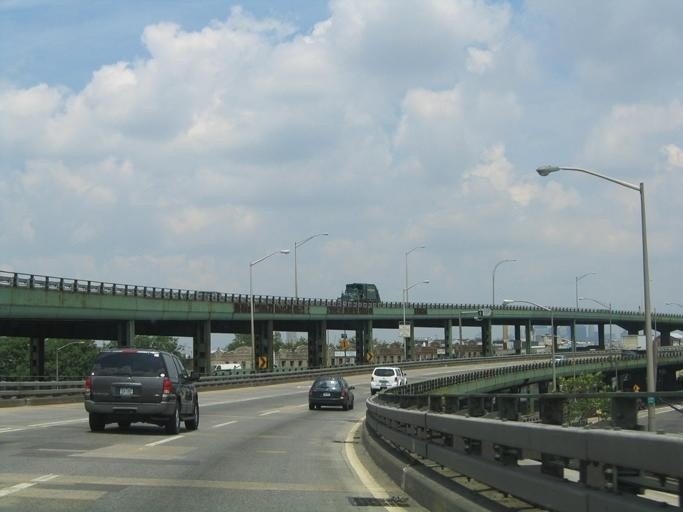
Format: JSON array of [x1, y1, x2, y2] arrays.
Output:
[[638, 398, 646, 411]]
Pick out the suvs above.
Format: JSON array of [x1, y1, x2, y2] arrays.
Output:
[[369, 367, 408, 396], [83, 345, 200, 435]]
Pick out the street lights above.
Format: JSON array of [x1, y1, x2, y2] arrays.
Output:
[[294, 232, 329, 302], [56, 341, 85, 391], [503, 298, 557, 395], [248, 248, 290, 373], [578, 297, 612, 361], [535, 162, 655, 436], [401, 245, 432, 360], [492, 258, 518, 307], [575, 271, 597, 312]]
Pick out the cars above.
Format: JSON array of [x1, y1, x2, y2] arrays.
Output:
[[308, 373, 355, 410]]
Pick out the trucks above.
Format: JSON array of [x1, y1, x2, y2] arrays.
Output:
[[345, 281, 382, 304], [622, 334, 654, 355]]
